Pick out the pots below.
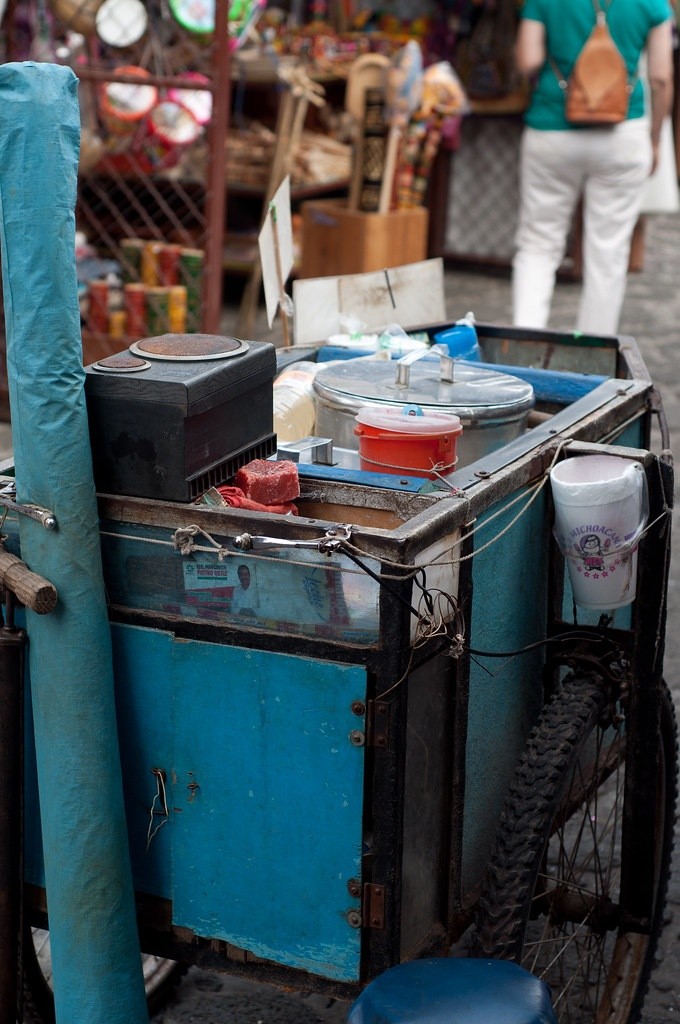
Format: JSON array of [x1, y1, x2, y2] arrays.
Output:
[[311, 349, 535, 473]]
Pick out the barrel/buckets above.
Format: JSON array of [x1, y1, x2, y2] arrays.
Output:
[[352, 403, 464, 482]]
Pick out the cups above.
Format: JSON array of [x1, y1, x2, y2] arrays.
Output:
[[550, 454, 642, 612]]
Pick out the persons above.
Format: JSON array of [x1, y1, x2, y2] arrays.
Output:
[[504, 0, 676, 345]]
